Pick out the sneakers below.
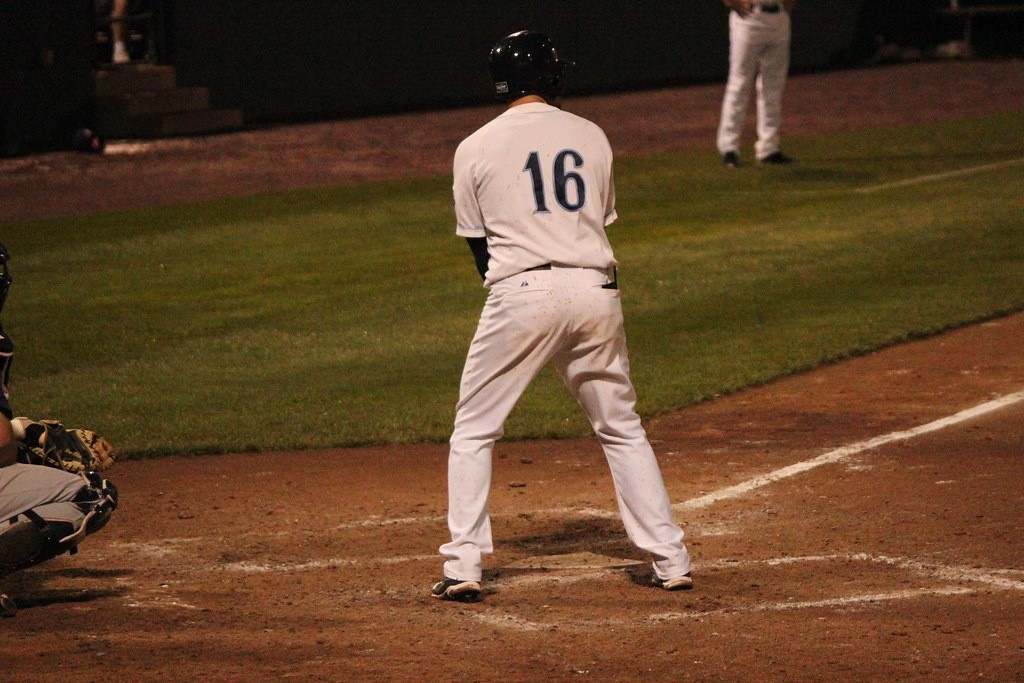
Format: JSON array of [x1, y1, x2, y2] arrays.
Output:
[[430, 578, 480, 599], [651, 572, 693, 589]]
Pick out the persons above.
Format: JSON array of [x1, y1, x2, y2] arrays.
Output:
[[0, 244, 119, 580], [428, 30, 692, 599], [716, 0, 795, 167]]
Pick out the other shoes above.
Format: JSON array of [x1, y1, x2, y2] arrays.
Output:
[[723, 152, 739, 168], [761, 152, 794, 166]]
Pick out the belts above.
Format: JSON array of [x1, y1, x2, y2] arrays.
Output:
[[748, 3, 781, 14], [522, 262, 595, 271]]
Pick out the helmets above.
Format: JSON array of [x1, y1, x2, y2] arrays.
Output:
[[487, 30, 576, 97]]
